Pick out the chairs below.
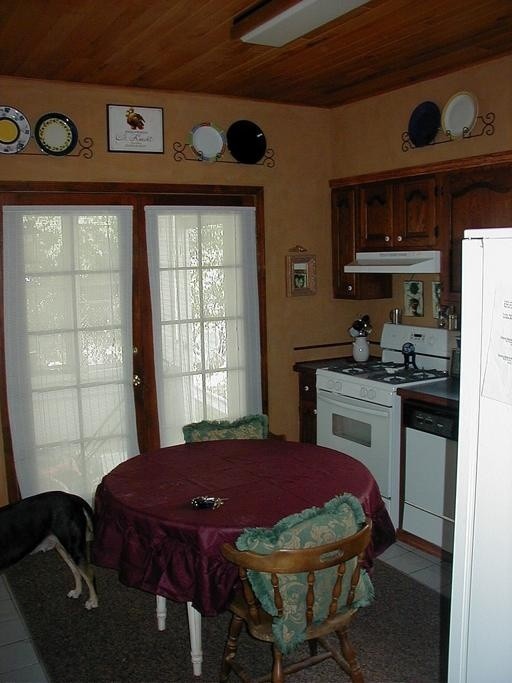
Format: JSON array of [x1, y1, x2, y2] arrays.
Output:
[[268, 432, 287, 440], [221, 516, 374, 683]]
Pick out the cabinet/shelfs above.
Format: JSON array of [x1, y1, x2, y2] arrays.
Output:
[[437, 150, 512, 307], [329, 169, 394, 300], [298, 371, 317, 446], [356, 161, 438, 252]]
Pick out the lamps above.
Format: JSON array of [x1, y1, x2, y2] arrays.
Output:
[[231, 0, 369, 48]]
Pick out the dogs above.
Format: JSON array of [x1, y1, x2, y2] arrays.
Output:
[[0, 491, 99, 610]]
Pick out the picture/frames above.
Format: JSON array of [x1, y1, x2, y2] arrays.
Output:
[[105, 104, 165, 154], [285, 255, 317, 297]]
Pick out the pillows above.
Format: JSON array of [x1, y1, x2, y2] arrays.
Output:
[[183, 413, 269, 443], [236, 492, 375, 655]]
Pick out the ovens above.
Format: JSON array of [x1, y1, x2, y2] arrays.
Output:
[[315, 391, 399, 524]]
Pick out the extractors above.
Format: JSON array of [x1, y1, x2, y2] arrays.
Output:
[[341, 251, 441, 276]]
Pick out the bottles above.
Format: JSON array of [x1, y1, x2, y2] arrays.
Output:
[[351, 336, 372, 362]]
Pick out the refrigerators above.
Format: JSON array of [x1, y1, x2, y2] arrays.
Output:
[[446, 225, 511, 682]]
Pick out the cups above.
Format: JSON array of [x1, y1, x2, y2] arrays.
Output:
[[449, 350, 460, 378], [390, 307, 400, 324]]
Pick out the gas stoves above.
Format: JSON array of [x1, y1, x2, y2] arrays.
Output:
[[315, 358, 448, 407]]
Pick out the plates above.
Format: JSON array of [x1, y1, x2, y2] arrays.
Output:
[[188, 120, 226, 163], [34, 112, 79, 157], [228, 121, 268, 163], [405, 102, 442, 146], [0, 104, 32, 154], [441, 90, 479, 136]]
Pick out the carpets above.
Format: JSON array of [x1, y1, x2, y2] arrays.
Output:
[[6, 537, 451, 682]]
